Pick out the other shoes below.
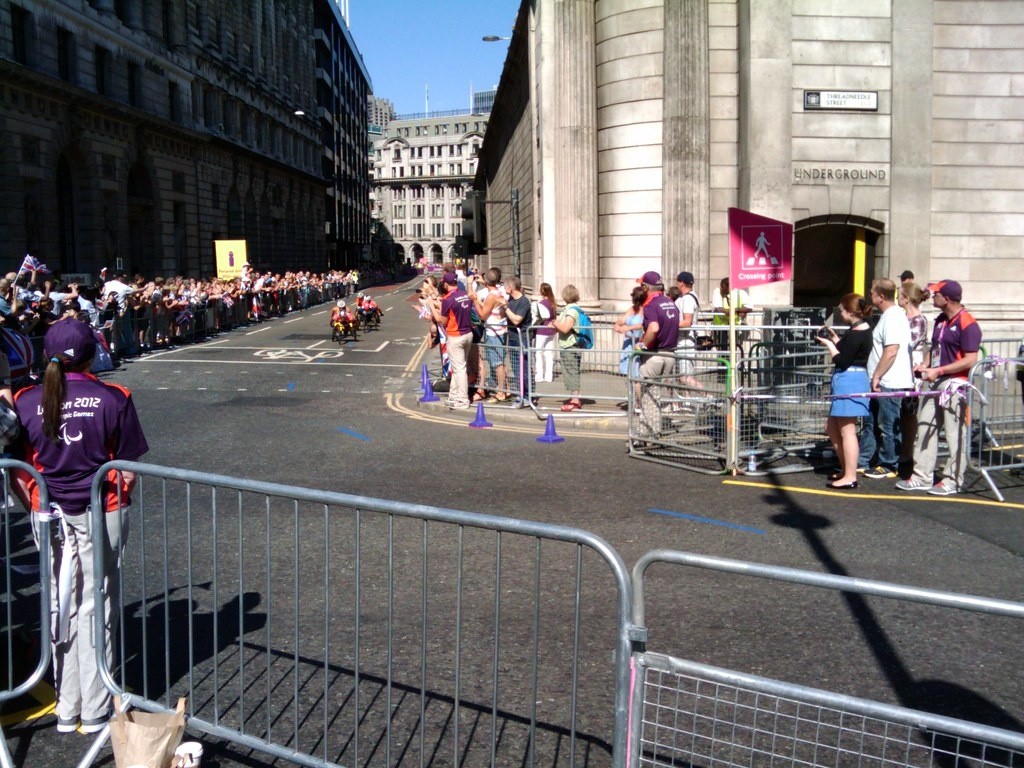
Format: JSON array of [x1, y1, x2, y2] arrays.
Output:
[[827, 473, 840, 480], [441, 376, 513, 409], [616, 401, 627, 409], [82, 711, 113, 733], [825, 481, 858, 489], [56, 714, 82, 733]]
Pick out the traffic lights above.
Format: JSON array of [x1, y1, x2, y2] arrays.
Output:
[[454, 189, 488, 259]]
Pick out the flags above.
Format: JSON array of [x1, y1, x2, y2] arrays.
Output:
[[19, 254, 51, 275]]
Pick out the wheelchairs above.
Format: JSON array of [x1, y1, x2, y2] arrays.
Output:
[[331, 311, 380, 345]]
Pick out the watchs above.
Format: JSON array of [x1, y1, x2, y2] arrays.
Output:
[[736, 307, 740, 312]]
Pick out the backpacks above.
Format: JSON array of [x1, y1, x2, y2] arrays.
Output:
[[569, 306, 593, 348]]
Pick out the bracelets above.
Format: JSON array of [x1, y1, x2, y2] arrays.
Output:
[[503, 306, 509, 311], [552, 320, 555, 325], [938, 367, 944, 376], [4, 379, 11, 385], [639, 342, 648, 350]]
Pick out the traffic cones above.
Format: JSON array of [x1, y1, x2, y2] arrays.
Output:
[[412, 364, 428, 393], [536, 414, 564, 443], [468, 402, 493, 428], [418, 379, 440, 402]]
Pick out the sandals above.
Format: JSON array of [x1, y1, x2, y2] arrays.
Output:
[[562, 402, 582, 411]]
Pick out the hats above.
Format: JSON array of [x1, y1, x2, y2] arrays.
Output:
[[440, 271, 458, 285], [927, 280, 962, 300], [44, 318, 97, 368], [676, 271, 693, 285], [636, 271, 662, 285]]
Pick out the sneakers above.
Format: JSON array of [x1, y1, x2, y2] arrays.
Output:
[[927, 477, 958, 496], [864, 464, 899, 478], [896, 478, 933, 490], [857, 467, 865, 474]]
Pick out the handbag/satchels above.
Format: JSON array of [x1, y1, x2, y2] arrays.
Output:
[[695, 336, 712, 350], [736, 323, 751, 340], [534, 304, 544, 326], [108, 694, 187, 768]]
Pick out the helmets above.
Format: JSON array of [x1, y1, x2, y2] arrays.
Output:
[[337, 300, 346, 307], [357, 292, 363, 298], [340, 311, 346, 317], [365, 296, 371, 301]]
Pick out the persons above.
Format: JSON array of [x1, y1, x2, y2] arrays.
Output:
[[357, 292, 383, 323], [612, 270, 754, 447], [353, 260, 458, 296], [814, 271, 981, 495], [0, 387, 18, 508], [1015, 339, 1024, 406], [549, 284, 583, 411], [429, 272, 473, 410], [0, 267, 243, 381], [497, 276, 537, 409], [469, 268, 510, 404], [234, 261, 359, 326], [530, 282, 556, 382], [411, 263, 479, 348], [12, 318, 150, 733], [328, 299, 358, 333]]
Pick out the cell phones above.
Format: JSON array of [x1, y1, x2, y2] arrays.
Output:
[[416, 288, 422, 293], [914, 371, 926, 379]]
